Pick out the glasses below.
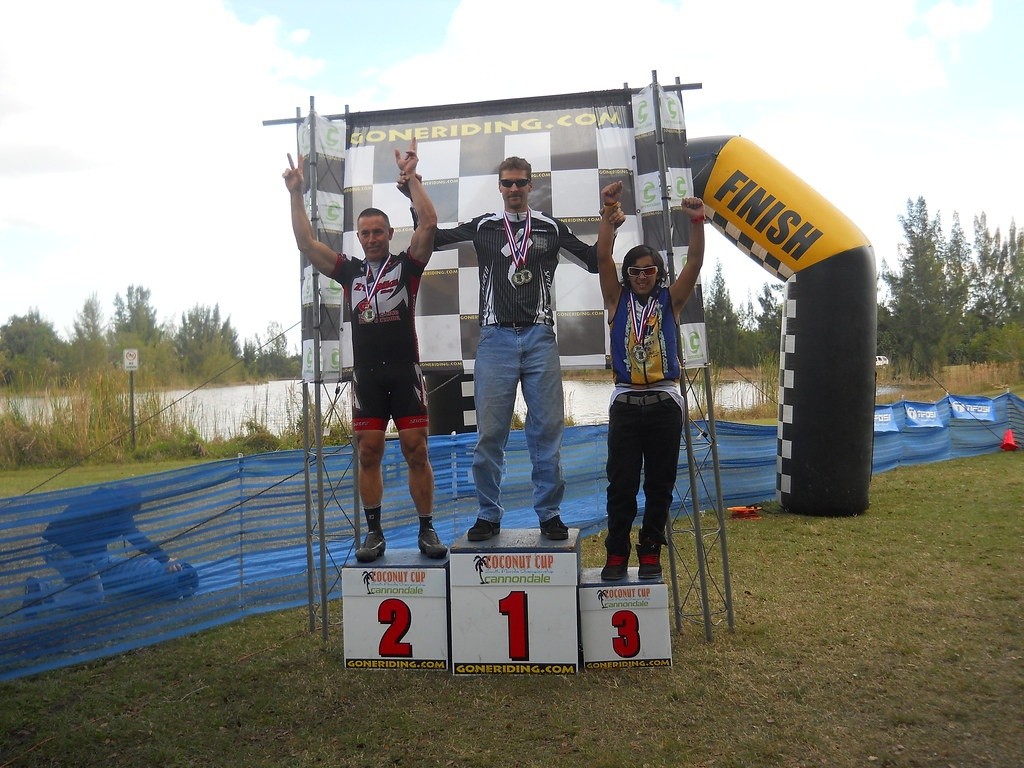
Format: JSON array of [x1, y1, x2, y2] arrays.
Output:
[[627, 266, 658, 278], [498, 179, 531, 187]]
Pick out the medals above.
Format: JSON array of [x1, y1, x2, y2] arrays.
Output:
[[361, 308, 375, 322], [512, 269, 532, 286], [633, 343, 647, 362]]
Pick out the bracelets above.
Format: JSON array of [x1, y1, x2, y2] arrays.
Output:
[[603, 202, 618, 207], [691, 215, 705, 223]]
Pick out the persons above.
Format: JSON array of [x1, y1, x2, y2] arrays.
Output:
[[397, 157, 627, 540], [597, 181, 706, 579], [282, 137, 448, 561], [22, 484, 182, 620]]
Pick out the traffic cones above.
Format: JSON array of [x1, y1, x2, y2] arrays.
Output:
[[1000, 429, 1018, 451]]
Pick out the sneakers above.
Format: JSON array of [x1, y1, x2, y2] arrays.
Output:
[[417, 530, 448, 559], [635, 543, 663, 578], [539, 515, 568, 540], [467, 518, 500, 541], [601, 549, 631, 580], [355, 536, 385, 562]]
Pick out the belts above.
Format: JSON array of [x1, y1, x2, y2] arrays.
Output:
[[615, 391, 672, 405], [491, 321, 533, 326]]
